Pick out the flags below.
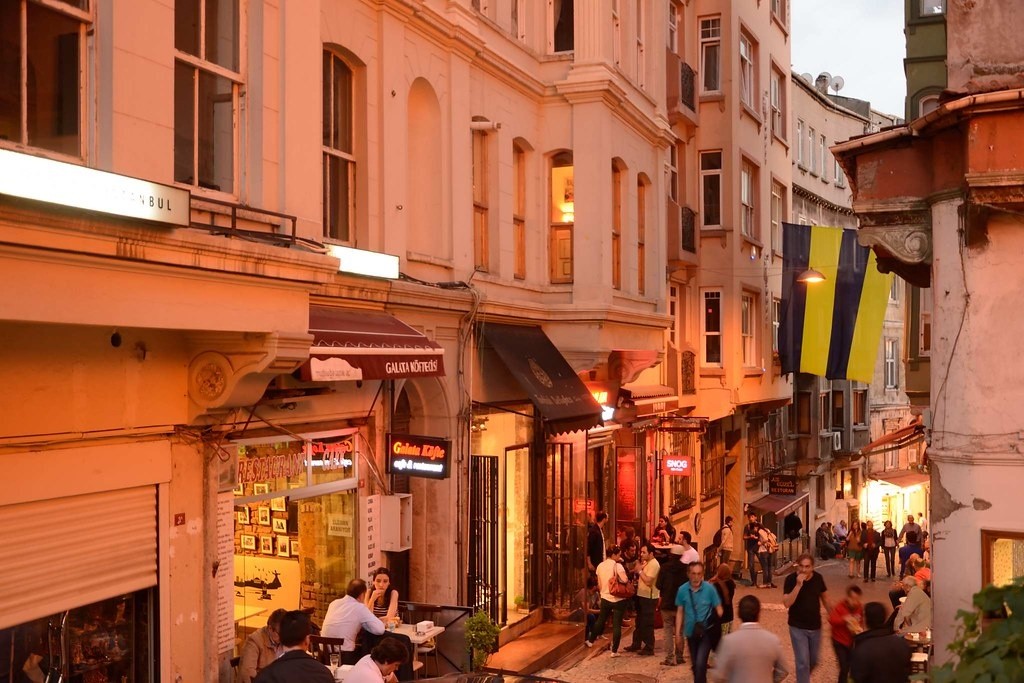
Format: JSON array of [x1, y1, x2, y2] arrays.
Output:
[[778, 221, 895, 384]]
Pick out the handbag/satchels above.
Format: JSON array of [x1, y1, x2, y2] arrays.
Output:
[[693, 621, 708, 640], [609, 564, 635, 598]]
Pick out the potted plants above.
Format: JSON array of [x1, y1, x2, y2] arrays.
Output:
[[514, 597, 525, 610], [456, 608, 504, 683]]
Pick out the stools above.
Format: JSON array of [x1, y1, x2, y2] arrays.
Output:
[[911, 652, 928, 675]]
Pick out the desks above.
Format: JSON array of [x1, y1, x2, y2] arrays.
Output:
[[234, 604, 268, 656], [904, 632, 931, 653], [651, 542, 682, 557], [383, 623, 445, 680]]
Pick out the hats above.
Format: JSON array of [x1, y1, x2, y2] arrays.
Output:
[[670, 545, 685, 556]]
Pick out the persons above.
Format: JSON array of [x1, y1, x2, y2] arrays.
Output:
[[816, 519, 848, 560], [827, 513, 933, 683], [743, 513, 778, 589], [234, 608, 336, 683], [711, 595, 790, 683], [570, 511, 733, 683], [341, 637, 408, 683], [782, 554, 832, 683], [319, 567, 399, 665]]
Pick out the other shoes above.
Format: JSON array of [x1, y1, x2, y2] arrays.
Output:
[[659, 660, 672, 665], [856, 573, 861, 578], [677, 660, 686, 664], [848, 574, 854, 578], [871, 577, 875, 581], [750, 582, 758, 588], [757, 584, 771, 588], [862, 579, 868, 583], [770, 582, 777, 589], [636, 649, 654, 656], [623, 645, 642, 651]]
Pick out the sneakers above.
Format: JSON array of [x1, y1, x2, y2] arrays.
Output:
[[585, 640, 593, 648], [611, 652, 621, 658]]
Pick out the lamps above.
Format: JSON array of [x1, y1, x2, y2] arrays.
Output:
[[471, 416, 488, 434], [796, 268, 825, 282]]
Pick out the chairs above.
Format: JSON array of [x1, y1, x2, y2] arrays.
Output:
[[230, 600, 442, 679]]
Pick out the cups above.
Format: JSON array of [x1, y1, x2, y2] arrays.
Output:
[[379, 616, 400, 630], [329, 654, 341, 680]]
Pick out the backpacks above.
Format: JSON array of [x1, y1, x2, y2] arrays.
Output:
[[762, 529, 779, 554], [713, 526, 729, 548]]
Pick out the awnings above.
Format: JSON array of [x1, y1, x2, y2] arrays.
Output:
[[477, 322, 605, 438], [293, 304, 447, 384]]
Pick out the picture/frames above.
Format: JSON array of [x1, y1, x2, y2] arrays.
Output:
[[233, 482, 299, 557]]
